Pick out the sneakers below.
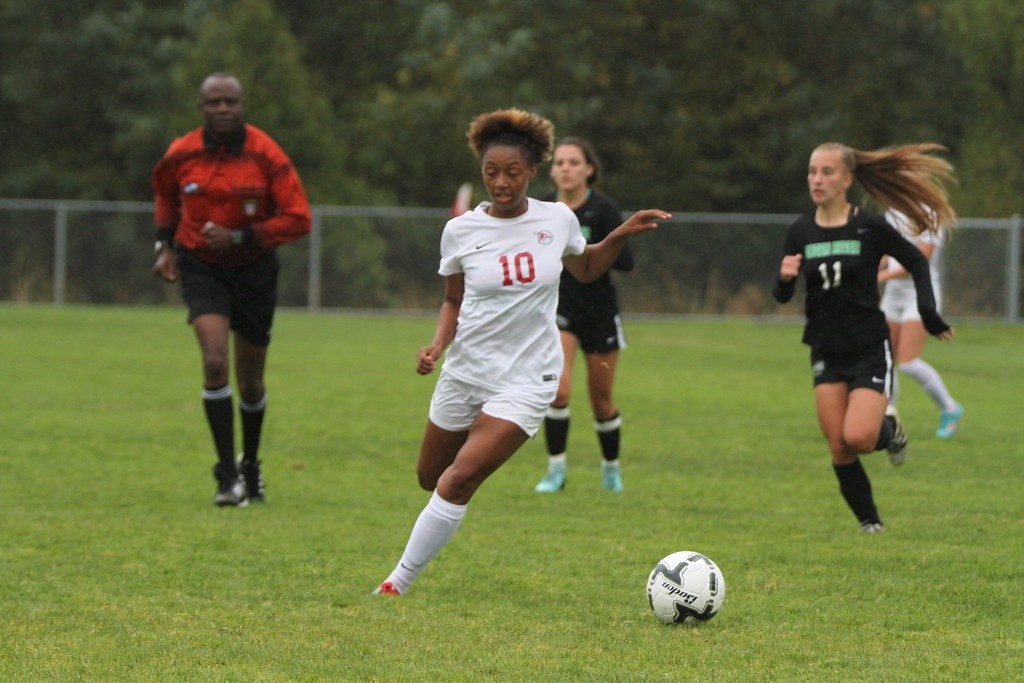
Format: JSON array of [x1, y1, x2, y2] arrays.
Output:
[[534, 463, 567, 493], [935, 402, 963, 437], [602, 463, 621, 493], [883, 405, 907, 467]]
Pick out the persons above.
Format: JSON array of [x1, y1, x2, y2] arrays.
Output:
[[877, 201, 966, 439], [150, 73, 310, 507], [373, 108, 671, 598], [772, 142, 952, 532], [538, 136, 636, 493]]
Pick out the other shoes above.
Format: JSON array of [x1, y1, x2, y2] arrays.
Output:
[[370, 582, 400, 596], [237, 451, 265, 507], [212, 462, 245, 504], [862, 521, 883, 534]]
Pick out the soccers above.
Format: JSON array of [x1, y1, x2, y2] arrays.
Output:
[[645, 550, 727, 628]]
